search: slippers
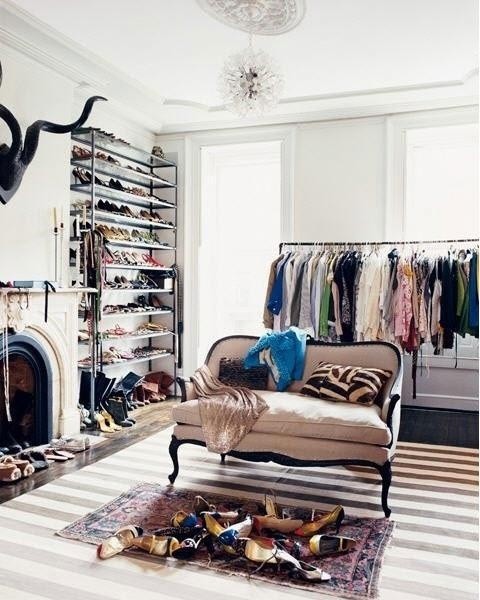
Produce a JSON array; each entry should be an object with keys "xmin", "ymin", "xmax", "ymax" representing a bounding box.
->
[{"xmin": 0, "ymin": 445, "xmax": 76, "ymax": 482}]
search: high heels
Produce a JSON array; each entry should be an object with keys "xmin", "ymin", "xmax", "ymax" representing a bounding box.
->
[
  {"xmin": 72, "ymin": 166, "xmax": 125, "ymax": 192},
  {"xmin": 131, "ymin": 487, "xmax": 357, "ymax": 582}
]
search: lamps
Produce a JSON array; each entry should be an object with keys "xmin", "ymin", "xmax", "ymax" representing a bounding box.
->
[{"xmin": 194, "ymin": 0, "xmax": 306, "ymax": 114}]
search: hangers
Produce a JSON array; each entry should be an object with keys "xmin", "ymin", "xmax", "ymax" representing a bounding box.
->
[{"xmin": 279, "ymin": 236, "xmax": 479, "ymax": 263}]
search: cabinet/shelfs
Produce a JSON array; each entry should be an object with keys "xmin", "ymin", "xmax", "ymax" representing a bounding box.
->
[{"xmin": 71, "ymin": 126, "xmax": 179, "ymax": 431}]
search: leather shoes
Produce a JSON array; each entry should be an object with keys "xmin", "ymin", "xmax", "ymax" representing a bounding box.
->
[
  {"xmin": 98, "ymin": 224, "xmax": 169, "ymax": 246},
  {"xmin": 93, "ymin": 127, "xmax": 131, "ymax": 145},
  {"xmin": 107, "ymin": 273, "xmax": 151, "ymax": 289},
  {"xmin": 98, "ymin": 199, "xmax": 166, "ymax": 224},
  {"xmin": 104, "ymin": 245, "xmax": 166, "ymax": 268}
]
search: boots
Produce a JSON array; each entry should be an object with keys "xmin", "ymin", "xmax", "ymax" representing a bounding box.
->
[{"xmin": 79, "ymin": 370, "xmax": 174, "ymax": 433}]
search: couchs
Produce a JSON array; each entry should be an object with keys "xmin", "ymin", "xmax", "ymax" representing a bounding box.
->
[{"xmin": 168, "ymin": 334, "xmax": 401, "ymax": 517}]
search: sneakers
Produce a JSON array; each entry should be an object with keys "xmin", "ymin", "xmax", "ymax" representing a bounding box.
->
[
  {"xmin": 60, "ymin": 435, "xmax": 91, "ymax": 448},
  {"xmin": 50, "ymin": 436, "xmax": 85, "ymax": 451}
]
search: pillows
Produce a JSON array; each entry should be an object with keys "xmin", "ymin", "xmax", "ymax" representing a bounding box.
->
[
  {"xmin": 300, "ymin": 361, "xmax": 392, "ymax": 407},
  {"xmin": 218, "ymin": 357, "xmax": 268, "ymax": 390}
]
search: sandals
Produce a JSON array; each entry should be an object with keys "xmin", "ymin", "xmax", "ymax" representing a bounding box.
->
[
  {"xmin": 96, "ymin": 524, "xmax": 143, "ymax": 560},
  {"xmin": 77, "ymin": 346, "xmax": 167, "ymax": 368},
  {"xmin": 71, "ymin": 145, "xmax": 109, "ymax": 162}
]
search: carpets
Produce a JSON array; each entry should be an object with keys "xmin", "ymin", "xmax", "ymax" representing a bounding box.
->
[{"xmin": 56, "ymin": 482, "xmax": 397, "ymax": 599}]
